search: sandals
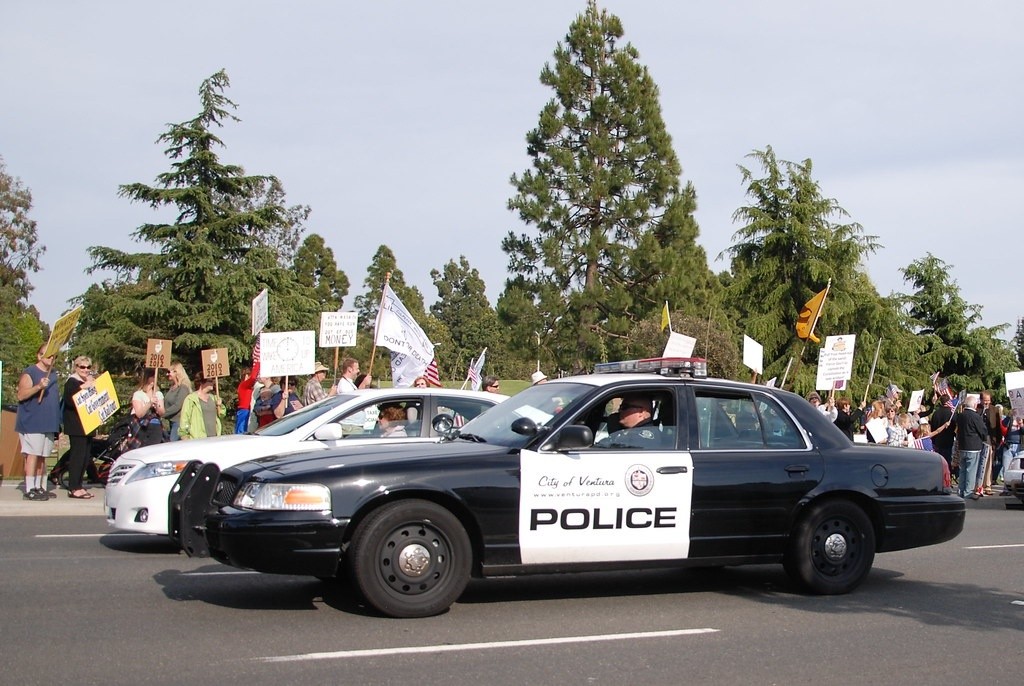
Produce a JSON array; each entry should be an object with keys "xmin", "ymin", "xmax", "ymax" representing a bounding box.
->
[{"xmin": 68, "ymin": 488, "xmax": 94, "ymax": 499}]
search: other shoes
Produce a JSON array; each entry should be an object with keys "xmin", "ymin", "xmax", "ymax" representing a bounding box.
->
[{"xmin": 959, "ymin": 482, "xmax": 1011, "ymax": 498}]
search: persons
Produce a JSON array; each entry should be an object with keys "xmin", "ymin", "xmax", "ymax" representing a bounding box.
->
[
  {"xmin": 377, "ymin": 402, "xmax": 409, "ymax": 438},
  {"xmin": 593, "ymin": 393, "xmax": 665, "ymax": 449},
  {"xmin": 480, "ymin": 371, "xmax": 565, "ymax": 417},
  {"xmin": 49, "ymin": 356, "xmax": 113, "ymax": 500},
  {"xmin": 133, "ymin": 371, "xmax": 165, "ymax": 445},
  {"xmin": 177, "ymin": 371, "xmax": 227, "ymax": 441},
  {"xmin": 154, "ymin": 361, "xmax": 194, "ymax": 442},
  {"xmin": 735, "ymin": 370, "xmax": 1024, "ymax": 501},
  {"xmin": 235, "ymin": 360, "xmax": 374, "ymax": 435},
  {"xmin": 14, "ymin": 342, "xmax": 61, "ymax": 501}
]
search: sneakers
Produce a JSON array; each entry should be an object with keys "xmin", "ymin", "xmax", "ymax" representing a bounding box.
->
[
  {"xmin": 36, "ymin": 487, "xmax": 57, "ymax": 498},
  {"xmin": 23, "ymin": 489, "xmax": 48, "ymax": 501}
]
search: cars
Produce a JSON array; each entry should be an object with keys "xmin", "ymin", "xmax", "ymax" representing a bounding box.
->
[
  {"xmin": 1004, "ymin": 451, "xmax": 1024, "ymax": 502},
  {"xmin": 97, "ymin": 388, "xmax": 609, "ymax": 536},
  {"xmin": 167, "ymin": 356, "xmax": 968, "ymax": 619}
]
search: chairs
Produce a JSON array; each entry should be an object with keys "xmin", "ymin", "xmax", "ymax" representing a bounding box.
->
[
  {"xmin": 607, "ymin": 399, "xmax": 786, "ymax": 450},
  {"xmin": 407, "ymin": 405, "xmax": 421, "ymax": 438}
]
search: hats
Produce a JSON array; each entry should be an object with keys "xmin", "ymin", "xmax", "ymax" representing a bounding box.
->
[
  {"xmin": 306, "ymin": 362, "xmax": 329, "ymax": 372},
  {"xmin": 530, "ymin": 370, "xmax": 548, "ymax": 386},
  {"xmin": 887, "ymin": 386, "xmax": 903, "ymax": 392}
]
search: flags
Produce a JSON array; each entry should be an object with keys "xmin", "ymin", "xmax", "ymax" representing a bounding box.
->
[
  {"xmin": 914, "ymin": 436, "xmax": 934, "ymax": 452},
  {"xmin": 938, "ymin": 379, "xmax": 948, "ymax": 391},
  {"xmin": 253, "ymin": 332, "xmax": 260, "ymax": 371},
  {"xmin": 929, "ymin": 372, "xmax": 941, "ymax": 383},
  {"xmin": 946, "ymin": 399, "xmax": 959, "ymax": 412},
  {"xmin": 796, "ymin": 288, "xmax": 828, "ymax": 343},
  {"xmin": 661, "ymin": 303, "xmax": 670, "ymax": 332},
  {"xmin": 375, "ymin": 280, "xmax": 486, "ymax": 428}
]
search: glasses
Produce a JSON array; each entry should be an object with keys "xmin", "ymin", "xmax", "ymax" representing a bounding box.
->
[
  {"xmin": 620, "ymin": 404, "xmax": 644, "ymax": 411},
  {"xmin": 166, "ymin": 370, "xmax": 171, "ymax": 373},
  {"xmin": 42, "ymin": 354, "xmax": 58, "ymax": 360},
  {"xmin": 77, "ymin": 364, "xmax": 92, "ymax": 369},
  {"xmin": 283, "ymin": 382, "xmax": 295, "ymax": 386},
  {"xmin": 378, "ymin": 413, "xmax": 384, "ymax": 419},
  {"xmin": 809, "ymin": 397, "xmax": 818, "ymax": 400},
  {"xmin": 490, "ymin": 385, "xmax": 499, "ymax": 388}
]
